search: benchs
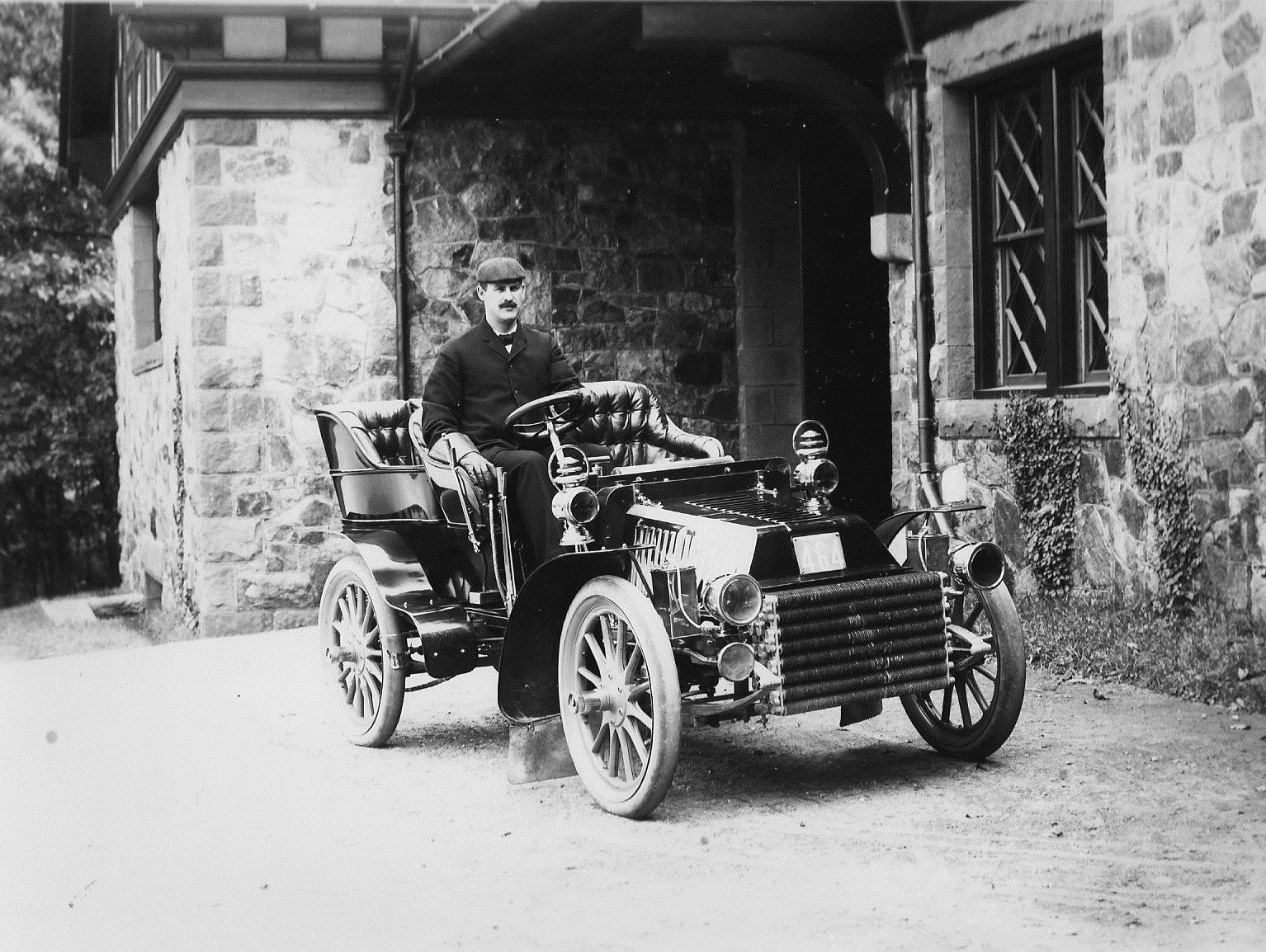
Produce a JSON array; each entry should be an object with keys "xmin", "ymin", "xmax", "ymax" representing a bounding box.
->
[{"xmin": 313, "ymin": 381, "xmax": 725, "ymax": 529}]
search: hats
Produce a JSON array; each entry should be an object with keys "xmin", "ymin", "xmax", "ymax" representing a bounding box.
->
[{"xmin": 476, "ymin": 257, "xmax": 525, "ymax": 282}]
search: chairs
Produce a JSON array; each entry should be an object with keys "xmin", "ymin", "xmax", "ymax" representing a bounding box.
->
[{"xmin": 407, "ymin": 406, "xmax": 528, "ymax": 616}]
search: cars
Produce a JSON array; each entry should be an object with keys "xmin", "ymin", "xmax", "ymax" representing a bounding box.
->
[{"xmin": 306, "ymin": 380, "xmax": 1028, "ymax": 822}]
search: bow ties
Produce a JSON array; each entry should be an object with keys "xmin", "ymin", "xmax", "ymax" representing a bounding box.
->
[{"xmin": 498, "ymin": 332, "xmax": 515, "ymax": 346}]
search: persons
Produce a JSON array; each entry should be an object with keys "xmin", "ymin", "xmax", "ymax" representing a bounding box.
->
[{"xmin": 422, "ymin": 258, "xmax": 615, "ymax": 568}]
